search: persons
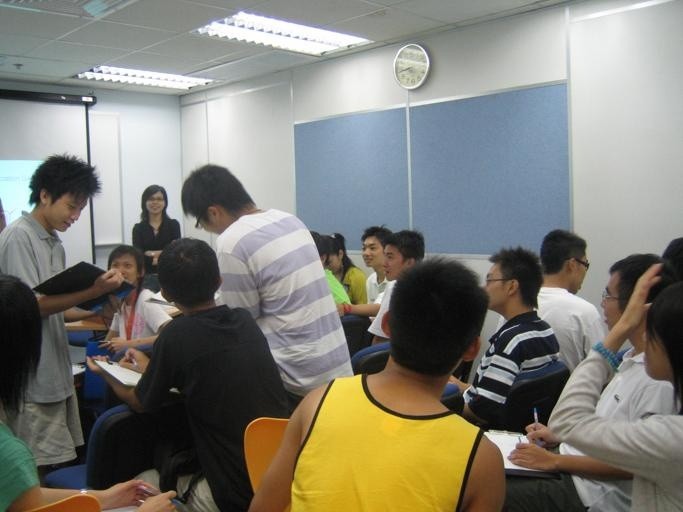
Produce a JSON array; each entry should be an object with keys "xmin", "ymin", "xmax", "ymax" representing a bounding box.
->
[
  {"xmin": 180, "ymin": 163, "xmax": 355, "ymax": 411},
  {"xmin": 132, "ymin": 184, "xmax": 182, "ymax": 297},
  {"xmin": 0, "ymin": 153, "xmax": 127, "ymax": 474},
  {"xmin": 1, "ymin": 225, "xmax": 679, "ymax": 511}
]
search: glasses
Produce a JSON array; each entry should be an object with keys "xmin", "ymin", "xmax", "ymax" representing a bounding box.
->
[
  {"xmin": 486, "ymin": 275, "xmax": 513, "ymax": 286},
  {"xmin": 602, "ymin": 290, "xmax": 618, "ymax": 302},
  {"xmin": 566, "ymin": 257, "xmax": 589, "ymax": 271}
]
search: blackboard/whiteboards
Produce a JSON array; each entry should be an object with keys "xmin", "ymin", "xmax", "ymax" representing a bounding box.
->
[{"xmin": 294, "ymin": 79, "xmax": 573, "ymax": 263}]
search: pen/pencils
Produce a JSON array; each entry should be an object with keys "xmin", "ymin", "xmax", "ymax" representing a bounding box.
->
[
  {"xmin": 138, "ymin": 486, "xmax": 182, "ymax": 505},
  {"xmin": 126, "ymin": 350, "xmax": 137, "ymax": 368},
  {"xmin": 534, "ymin": 408, "xmax": 541, "ymax": 446},
  {"xmin": 93, "ymin": 330, "xmax": 97, "ymax": 341}
]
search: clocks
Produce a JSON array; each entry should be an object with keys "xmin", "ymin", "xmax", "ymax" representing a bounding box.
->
[{"xmin": 392, "ymin": 44, "xmax": 430, "ymax": 90}]
